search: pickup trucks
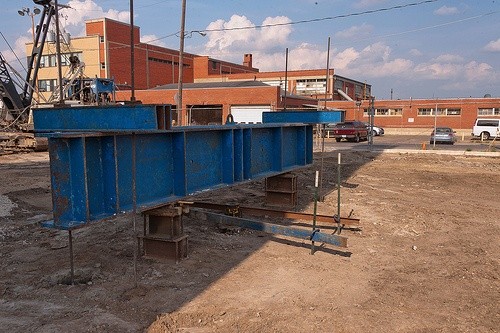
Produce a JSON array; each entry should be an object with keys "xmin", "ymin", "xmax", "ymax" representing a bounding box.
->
[{"xmin": 333, "ymin": 120, "xmax": 370, "ymax": 144}]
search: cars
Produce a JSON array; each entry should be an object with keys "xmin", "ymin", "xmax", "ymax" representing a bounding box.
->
[{"xmin": 430, "ymin": 126, "xmax": 457, "ymax": 144}]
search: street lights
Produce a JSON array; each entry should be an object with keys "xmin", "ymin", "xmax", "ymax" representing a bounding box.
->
[
  {"xmin": 17, "ymin": 6, "xmax": 43, "ymax": 109},
  {"xmin": 370, "ymin": 93, "xmax": 376, "ymax": 144}
]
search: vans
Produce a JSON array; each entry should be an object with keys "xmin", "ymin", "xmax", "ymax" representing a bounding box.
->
[{"xmin": 471, "ymin": 118, "xmax": 500, "ymax": 141}]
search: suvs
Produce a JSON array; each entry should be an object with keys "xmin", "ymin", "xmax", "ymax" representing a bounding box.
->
[{"xmin": 363, "ymin": 122, "xmax": 384, "ymax": 137}]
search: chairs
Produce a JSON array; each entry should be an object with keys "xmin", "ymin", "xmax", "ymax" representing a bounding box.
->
[
  {"xmin": 437, "ymin": 130, "xmax": 441, "ymax": 132},
  {"xmin": 446, "ymin": 130, "xmax": 449, "ymax": 132}
]
